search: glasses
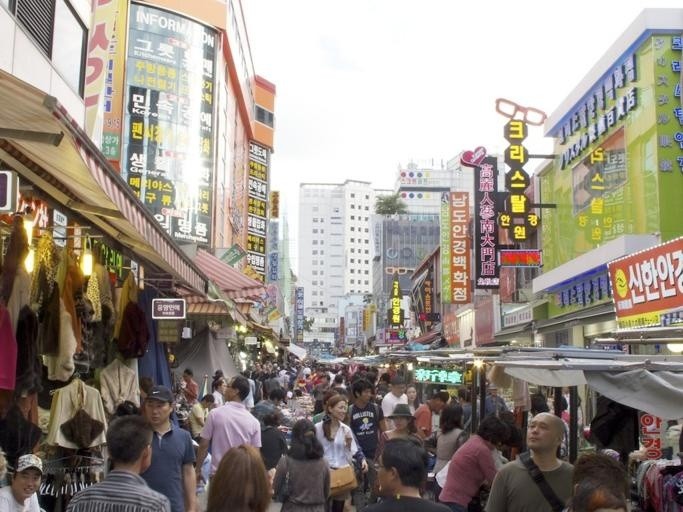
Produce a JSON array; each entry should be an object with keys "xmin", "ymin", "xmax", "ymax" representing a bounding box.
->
[
  {"xmin": 373, "ymin": 463, "xmax": 385, "ymax": 471},
  {"xmin": 223, "ymin": 384, "xmax": 232, "ymax": 388}
]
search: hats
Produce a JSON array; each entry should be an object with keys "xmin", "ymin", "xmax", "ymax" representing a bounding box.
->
[
  {"xmin": 15, "ymin": 454, "xmax": 46, "ymax": 474},
  {"xmin": 385, "ymin": 404, "xmax": 415, "ymax": 419},
  {"xmin": 488, "ymin": 382, "xmax": 495, "ymax": 390},
  {"xmin": 145, "ymin": 385, "xmax": 174, "ymax": 402},
  {"xmin": 440, "ymin": 389, "xmax": 452, "ymax": 405}
]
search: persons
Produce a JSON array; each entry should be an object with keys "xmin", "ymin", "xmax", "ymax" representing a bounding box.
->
[
  {"xmin": 196, "ymin": 375, "xmax": 262, "ymax": 493},
  {"xmin": 138, "ymin": 377, "xmax": 153, "ymax": 402},
  {"xmin": 381, "ymin": 376, "xmax": 408, "ymax": 430},
  {"xmin": 344, "ymin": 359, "xmax": 413, "ymax": 384},
  {"xmin": 250, "ymin": 387, "xmax": 284, "ymax": 428},
  {"xmin": 272, "ymin": 418, "xmax": 331, "ymax": 512},
  {"xmin": 206, "ymin": 443, "xmax": 273, "ymax": 512},
  {"xmin": 449, "ymin": 382, "xmax": 483, "ymax": 424},
  {"xmin": 1, "ymin": 455, "xmax": 43, "ymax": 511},
  {"xmin": 552, "ymin": 396, "xmax": 570, "ymax": 424},
  {"xmin": 484, "ymin": 412, "xmax": 574, "ymax": 512},
  {"xmin": 563, "ymin": 452, "xmax": 629, "ymax": 512},
  {"xmin": 138, "ymin": 385, "xmax": 196, "ymax": 512},
  {"xmin": 414, "ymin": 391, "xmax": 449, "ymax": 436},
  {"xmin": 484, "ymin": 383, "xmax": 510, "ymax": 414},
  {"xmin": 528, "ymin": 392, "xmax": 551, "ymax": 418},
  {"xmin": 589, "ymin": 415, "xmax": 623, "ymax": 464},
  {"xmin": 358, "ymin": 437, "xmax": 452, "ymax": 511},
  {"xmin": 314, "ymin": 395, "xmax": 357, "ymax": 512},
  {"xmin": 259, "ymin": 407, "xmax": 290, "ymax": 474},
  {"xmin": 347, "ymin": 381, "xmax": 387, "ymax": 512},
  {"xmin": 234, "ymin": 354, "xmax": 351, "ymax": 394},
  {"xmin": 191, "ymin": 394, "xmax": 215, "ymax": 444},
  {"xmin": 67, "ymin": 415, "xmax": 171, "ymax": 512},
  {"xmin": 115, "ymin": 401, "xmax": 139, "ymax": 419},
  {"xmin": 405, "ymin": 383, "xmax": 418, "ymax": 415},
  {"xmin": 375, "ymin": 404, "xmax": 425, "ymax": 465},
  {"xmin": 212, "ymin": 368, "xmax": 225, "ymax": 395},
  {"xmin": 181, "ymin": 368, "xmax": 200, "ymax": 409},
  {"xmin": 438, "ymin": 415, "xmax": 511, "ymax": 512},
  {"xmin": 212, "ymin": 377, "xmax": 226, "ymax": 409},
  {"xmin": 431, "ymin": 402, "xmax": 469, "ymax": 473}
]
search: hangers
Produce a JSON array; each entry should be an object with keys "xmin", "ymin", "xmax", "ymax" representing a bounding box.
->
[
  {"xmin": 73, "ymin": 372, "xmax": 81, "ymax": 379},
  {"xmin": 39, "ymin": 465, "xmax": 106, "ymax": 497}
]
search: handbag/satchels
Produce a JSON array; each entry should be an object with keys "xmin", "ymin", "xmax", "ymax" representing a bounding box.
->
[
  {"xmin": 270, "ymin": 459, "xmax": 291, "ymax": 506},
  {"xmin": 521, "ymin": 451, "xmax": 562, "ymax": 512},
  {"xmin": 325, "ymin": 464, "xmax": 358, "ymax": 494}
]
